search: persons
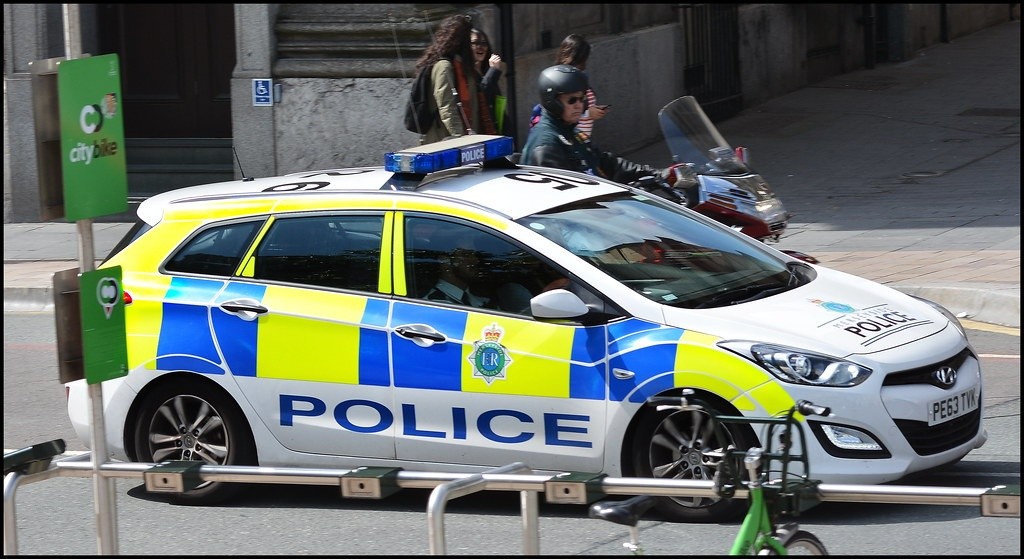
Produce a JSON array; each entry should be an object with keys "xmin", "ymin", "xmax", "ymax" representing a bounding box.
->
[
  {"xmin": 529, "ymin": 34, "xmax": 610, "ymax": 145},
  {"xmin": 418, "ymin": 229, "xmax": 569, "ymax": 315},
  {"xmin": 417, "ymin": 14, "xmax": 479, "ymax": 145},
  {"xmin": 519, "ymin": 64, "xmax": 695, "ymax": 195},
  {"xmin": 469, "ymin": 28, "xmax": 505, "ymax": 138}
]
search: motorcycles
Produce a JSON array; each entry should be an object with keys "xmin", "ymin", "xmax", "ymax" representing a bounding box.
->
[{"xmin": 626, "ymin": 96, "xmax": 821, "ymax": 264}]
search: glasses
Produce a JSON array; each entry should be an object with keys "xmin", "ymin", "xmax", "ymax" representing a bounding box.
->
[
  {"xmin": 560, "ymin": 94, "xmax": 587, "ymax": 105},
  {"xmin": 472, "ymin": 41, "xmax": 487, "ymax": 46}
]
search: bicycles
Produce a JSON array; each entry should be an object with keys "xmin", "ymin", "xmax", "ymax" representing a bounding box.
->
[{"xmin": 585, "ymin": 389, "xmax": 830, "ymax": 556}]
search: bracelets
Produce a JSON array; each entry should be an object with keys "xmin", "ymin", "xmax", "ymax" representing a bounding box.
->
[{"xmin": 584, "ymin": 110, "xmax": 590, "ymax": 119}]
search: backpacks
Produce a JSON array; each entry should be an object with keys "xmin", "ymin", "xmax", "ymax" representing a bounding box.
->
[{"xmin": 405, "ymin": 63, "xmax": 442, "ymax": 134}]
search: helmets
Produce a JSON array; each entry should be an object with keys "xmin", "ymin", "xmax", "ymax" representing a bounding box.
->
[{"xmin": 538, "ymin": 64, "xmax": 590, "ymax": 119}]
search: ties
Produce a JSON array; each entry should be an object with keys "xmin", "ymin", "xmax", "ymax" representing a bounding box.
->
[{"xmin": 462, "ymin": 292, "xmax": 469, "ymax": 304}]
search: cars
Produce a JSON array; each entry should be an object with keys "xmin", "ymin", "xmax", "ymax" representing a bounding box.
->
[{"xmin": 64, "ymin": 133, "xmax": 989, "ymax": 525}]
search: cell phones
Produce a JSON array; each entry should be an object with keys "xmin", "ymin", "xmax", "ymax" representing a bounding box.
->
[{"xmin": 602, "ymin": 104, "xmax": 611, "ymax": 110}]
